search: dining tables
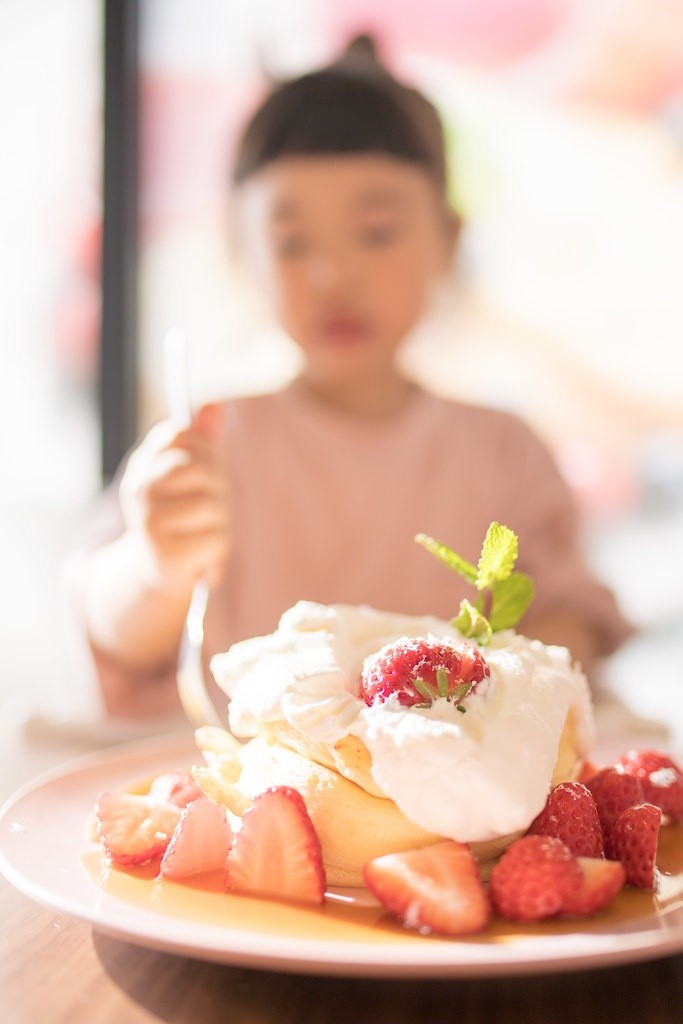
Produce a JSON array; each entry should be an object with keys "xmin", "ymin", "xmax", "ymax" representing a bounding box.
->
[{"xmin": 0, "ymin": 717, "xmax": 683, "ymax": 1024}]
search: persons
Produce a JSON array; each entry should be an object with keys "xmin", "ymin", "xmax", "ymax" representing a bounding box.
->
[{"xmin": 73, "ymin": 42, "xmax": 631, "ymax": 720}]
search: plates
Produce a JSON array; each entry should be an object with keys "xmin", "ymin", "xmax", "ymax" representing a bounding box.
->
[{"xmin": 0, "ymin": 730, "xmax": 681, "ymax": 978}]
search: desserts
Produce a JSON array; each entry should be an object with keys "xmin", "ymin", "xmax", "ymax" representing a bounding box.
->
[{"xmin": 188, "ymin": 519, "xmax": 597, "ymax": 886}]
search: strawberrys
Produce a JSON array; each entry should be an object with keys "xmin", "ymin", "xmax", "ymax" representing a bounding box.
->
[
  {"xmin": 361, "ymin": 750, "xmax": 682, "ymax": 935},
  {"xmin": 89, "ymin": 772, "xmax": 326, "ymax": 906}
]
